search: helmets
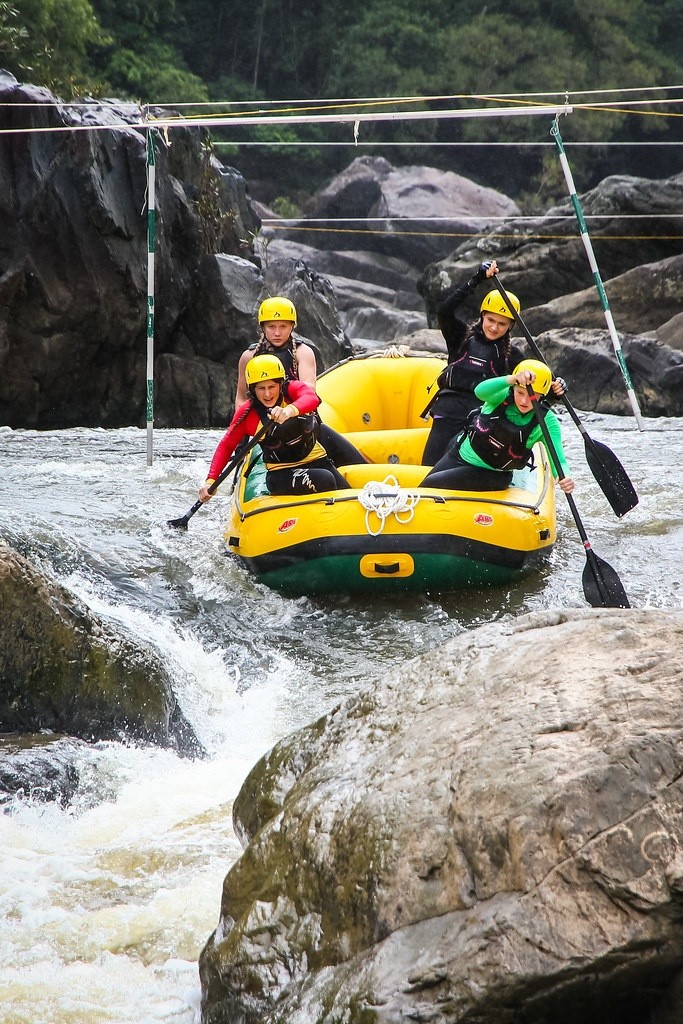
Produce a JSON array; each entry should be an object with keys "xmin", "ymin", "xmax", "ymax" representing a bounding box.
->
[
  {"xmin": 245, "ymin": 354, "xmax": 285, "ymax": 388},
  {"xmin": 512, "ymin": 359, "xmax": 552, "ymax": 396},
  {"xmin": 258, "ymin": 297, "xmax": 297, "ymax": 325},
  {"xmin": 480, "ymin": 289, "xmax": 520, "ymax": 320}
]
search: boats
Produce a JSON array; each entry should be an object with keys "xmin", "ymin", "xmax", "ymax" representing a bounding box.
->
[{"xmin": 220, "ymin": 344, "xmax": 566, "ymax": 595}]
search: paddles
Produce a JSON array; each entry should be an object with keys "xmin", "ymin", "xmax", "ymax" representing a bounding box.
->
[
  {"xmin": 524, "ymin": 382, "xmax": 630, "ymax": 607},
  {"xmin": 166, "ymin": 419, "xmax": 274, "ymax": 530},
  {"xmin": 490, "ymin": 269, "xmax": 639, "ymax": 518},
  {"xmin": 228, "ymin": 433, "xmax": 250, "ymax": 494}
]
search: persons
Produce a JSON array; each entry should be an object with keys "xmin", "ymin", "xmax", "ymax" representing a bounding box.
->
[
  {"xmin": 197, "ymin": 356, "xmax": 353, "ymax": 503},
  {"xmin": 419, "ymin": 261, "xmax": 569, "ymax": 468},
  {"xmin": 233, "ymin": 296, "xmax": 367, "ymax": 468},
  {"xmin": 416, "ymin": 358, "xmax": 576, "ymax": 494}
]
